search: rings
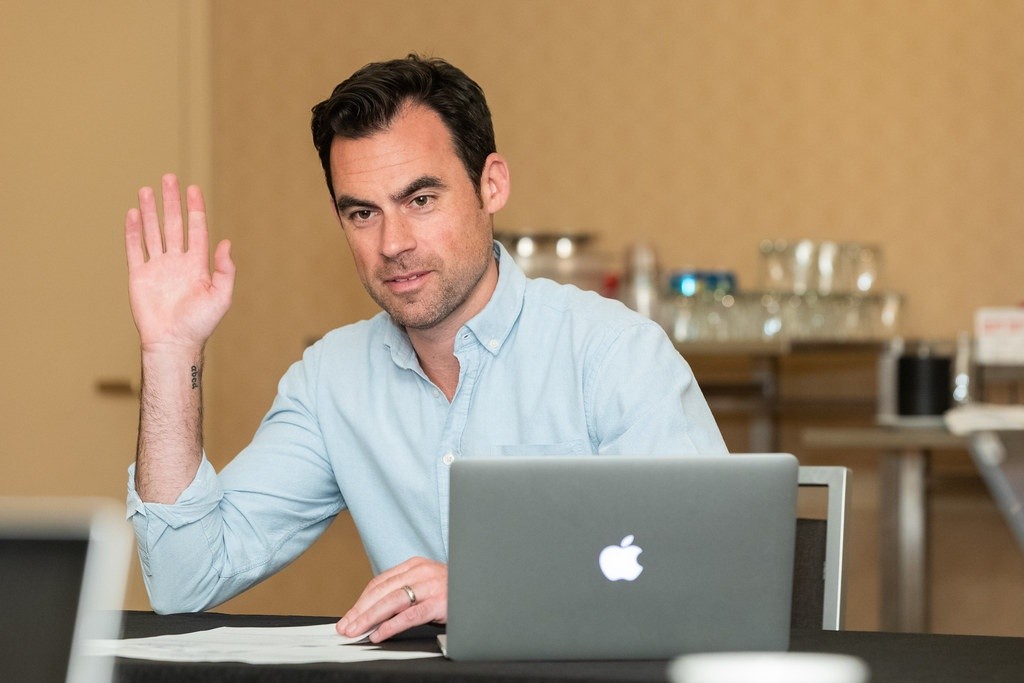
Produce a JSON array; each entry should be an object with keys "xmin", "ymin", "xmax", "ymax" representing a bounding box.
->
[{"xmin": 400, "ymin": 584, "xmax": 417, "ymax": 607}]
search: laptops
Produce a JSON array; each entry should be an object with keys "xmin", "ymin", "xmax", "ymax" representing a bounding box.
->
[{"xmin": 439, "ymin": 452, "xmax": 798, "ymax": 661}]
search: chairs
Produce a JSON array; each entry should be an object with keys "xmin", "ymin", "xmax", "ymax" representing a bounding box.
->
[{"xmin": 788, "ymin": 465, "xmax": 854, "ymax": 632}]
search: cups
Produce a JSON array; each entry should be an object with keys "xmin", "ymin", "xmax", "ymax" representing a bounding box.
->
[{"xmin": 898, "ymin": 354, "xmax": 952, "ymax": 416}]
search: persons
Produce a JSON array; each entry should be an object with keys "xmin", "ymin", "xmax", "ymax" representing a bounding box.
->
[{"xmin": 123, "ymin": 53, "xmax": 732, "ymax": 644}]
